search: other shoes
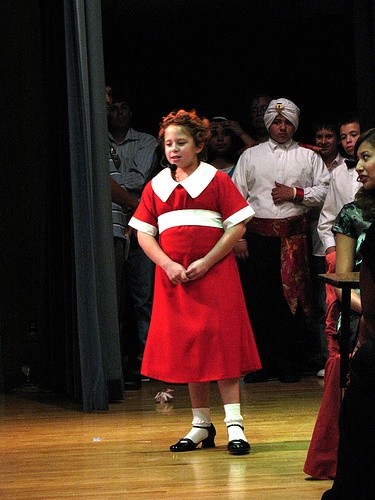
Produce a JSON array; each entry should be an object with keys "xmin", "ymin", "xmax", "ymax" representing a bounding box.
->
[
  {"xmin": 311, "ymin": 362, "xmax": 327, "ymax": 379},
  {"xmin": 322, "ymin": 489, "xmax": 339, "ymax": 500},
  {"xmin": 277, "ymin": 366, "xmax": 297, "ymax": 383},
  {"xmin": 244, "ymin": 368, "xmax": 278, "ymax": 382}
]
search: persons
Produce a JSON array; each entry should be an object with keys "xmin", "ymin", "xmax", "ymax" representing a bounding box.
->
[{"xmin": 96, "ymin": 82, "xmax": 374, "ymax": 500}]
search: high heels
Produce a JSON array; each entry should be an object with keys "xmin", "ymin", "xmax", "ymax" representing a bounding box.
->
[
  {"xmin": 227, "ymin": 424, "xmax": 250, "ymax": 453},
  {"xmin": 169, "ymin": 423, "xmax": 216, "ymax": 453}
]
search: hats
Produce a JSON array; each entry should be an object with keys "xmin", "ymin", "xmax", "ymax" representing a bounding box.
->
[{"xmin": 263, "ymin": 98, "xmax": 300, "ymax": 133}]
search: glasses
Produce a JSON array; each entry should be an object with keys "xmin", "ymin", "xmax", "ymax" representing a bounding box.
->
[{"xmin": 249, "ymin": 103, "xmax": 267, "ymax": 113}]
